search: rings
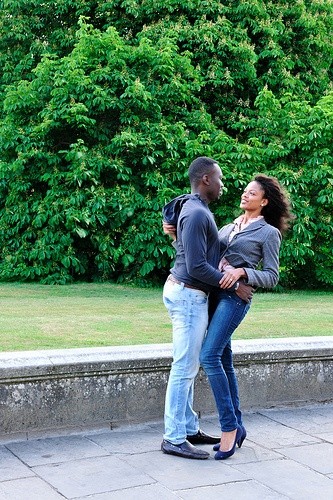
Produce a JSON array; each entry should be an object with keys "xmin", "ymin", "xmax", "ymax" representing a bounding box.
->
[{"xmin": 230, "ymin": 279, "xmax": 233, "ymax": 282}]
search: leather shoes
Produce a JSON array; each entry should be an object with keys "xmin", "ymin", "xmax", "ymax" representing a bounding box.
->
[
  {"xmin": 160, "ymin": 438, "xmax": 210, "ymax": 460},
  {"xmin": 188, "ymin": 432, "xmax": 222, "ymax": 444}
]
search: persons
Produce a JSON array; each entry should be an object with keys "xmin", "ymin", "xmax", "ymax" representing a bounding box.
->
[
  {"xmin": 161, "ymin": 172, "xmax": 296, "ymax": 462},
  {"xmin": 160, "ymin": 155, "xmax": 256, "ymax": 461}
]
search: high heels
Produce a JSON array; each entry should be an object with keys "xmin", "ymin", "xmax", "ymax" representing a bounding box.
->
[
  {"xmin": 214, "ymin": 425, "xmax": 242, "ymax": 459},
  {"xmin": 214, "ymin": 424, "xmax": 246, "ymax": 449}
]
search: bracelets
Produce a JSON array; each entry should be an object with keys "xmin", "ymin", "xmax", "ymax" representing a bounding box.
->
[{"xmin": 235, "ymin": 282, "xmax": 239, "ymax": 290}]
